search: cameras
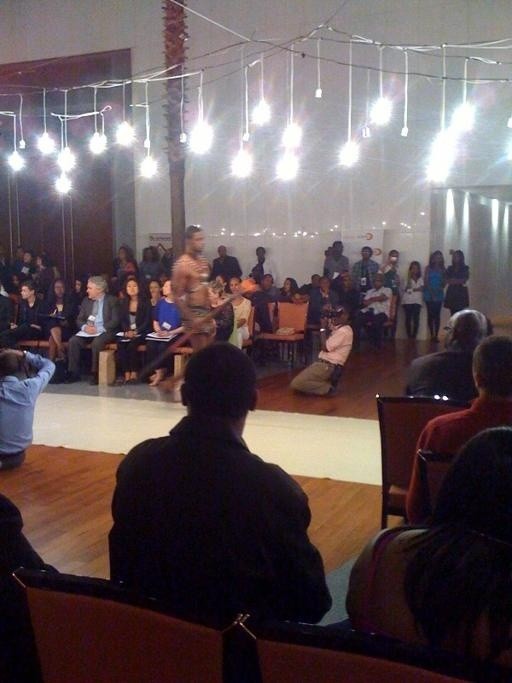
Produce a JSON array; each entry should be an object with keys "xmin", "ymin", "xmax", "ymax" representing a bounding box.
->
[{"xmin": 320, "ymin": 310, "xmax": 338, "ymax": 318}]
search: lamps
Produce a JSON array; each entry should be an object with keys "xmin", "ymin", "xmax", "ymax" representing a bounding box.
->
[{"xmin": 0, "ymin": 5, "xmax": 512, "ymax": 195}]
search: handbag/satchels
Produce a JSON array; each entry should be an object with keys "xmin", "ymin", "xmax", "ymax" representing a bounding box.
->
[{"xmin": 48, "ymin": 358, "xmax": 68, "ymax": 383}]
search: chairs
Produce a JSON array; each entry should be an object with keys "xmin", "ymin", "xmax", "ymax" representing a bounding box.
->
[
  {"xmin": 376, "ymin": 392, "xmax": 473, "ymax": 533},
  {"xmin": 415, "ymin": 449, "xmax": 456, "ymax": 522},
  {"xmin": 243, "ymin": 607, "xmax": 512, "ymax": 683},
  {"xmin": 2, "ymin": 273, "xmax": 398, "ymax": 388},
  {"xmin": 11, "ymin": 562, "xmax": 243, "ymax": 682}
]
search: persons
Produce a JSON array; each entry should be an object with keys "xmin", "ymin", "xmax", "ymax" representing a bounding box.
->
[
  {"xmin": 209, "ymin": 242, "xmax": 404, "ymax": 351},
  {"xmin": 0, "ymin": 246, "xmax": 184, "ymax": 385},
  {"xmin": 444, "ymin": 250, "xmax": 468, "ymax": 316},
  {"xmin": 0, "ymin": 490, "xmax": 61, "ymax": 683},
  {"xmin": 405, "ymin": 335, "xmax": 512, "ymax": 526},
  {"xmin": 157, "ymin": 226, "xmax": 217, "ymax": 396},
  {"xmin": 0, "ymin": 347, "xmax": 56, "ymax": 469},
  {"xmin": 405, "ymin": 307, "xmax": 492, "ymax": 400},
  {"xmin": 343, "ymin": 426, "xmax": 512, "ymax": 682},
  {"xmin": 402, "ymin": 261, "xmax": 423, "ymax": 341},
  {"xmin": 422, "ymin": 251, "xmax": 447, "ymax": 342},
  {"xmin": 109, "ymin": 340, "xmax": 333, "ymax": 637},
  {"xmin": 290, "ymin": 305, "xmax": 353, "ymax": 397}
]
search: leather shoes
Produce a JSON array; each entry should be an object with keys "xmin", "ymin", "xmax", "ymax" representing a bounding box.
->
[{"xmin": 123, "ymin": 374, "xmax": 165, "ymax": 385}]
